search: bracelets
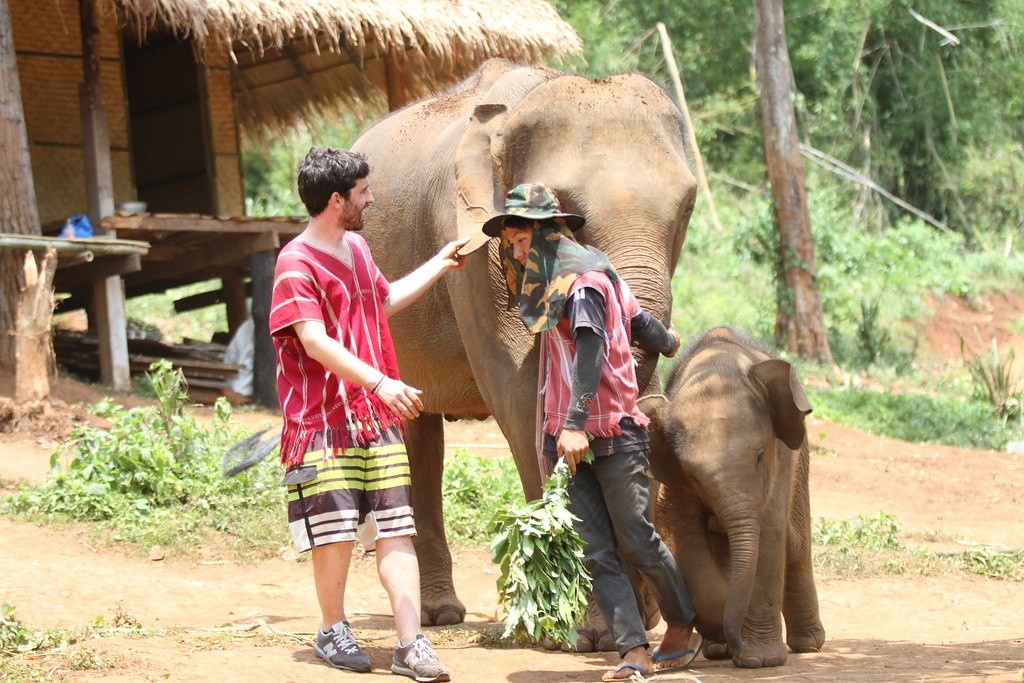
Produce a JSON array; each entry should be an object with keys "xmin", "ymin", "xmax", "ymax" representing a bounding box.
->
[{"xmin": 371, "ymin": 375, "xmax": 386, "ymax": 395}]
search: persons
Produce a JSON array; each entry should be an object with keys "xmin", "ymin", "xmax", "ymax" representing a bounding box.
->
[
  {"xmin": 270, "ymin": 147, "xmax": 470, "ymax": 681},
  {"xmin": 481, "ymin": 185, "xmax": 702, "ymax": 682}
]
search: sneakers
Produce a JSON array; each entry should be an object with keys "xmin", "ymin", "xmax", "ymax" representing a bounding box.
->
[
  {"xmin": 314, "ymin": 618, "xmax": 372, "ymax": 672},
  {"xmin": 390, "ymin": 632, "xmax": 450, "ymax": 682}
]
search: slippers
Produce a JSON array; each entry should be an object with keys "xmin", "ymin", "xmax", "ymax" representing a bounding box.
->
[
  {"xmin": 602, "ymin": 663, "xmax": 656, "ymax": 681},
  {"xmin": 649, "ymin": 632, "xmax": 703, "ymax": 673}
]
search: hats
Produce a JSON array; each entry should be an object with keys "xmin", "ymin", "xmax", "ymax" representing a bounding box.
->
[{"xmin": 482, "ymin": 182, "xmax": 586, "ymax": 237}]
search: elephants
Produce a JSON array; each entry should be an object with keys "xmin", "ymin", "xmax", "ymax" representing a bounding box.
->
[{"xmin": 347, "ymin": 58, "xmax": 825, "ymax": 667}]
